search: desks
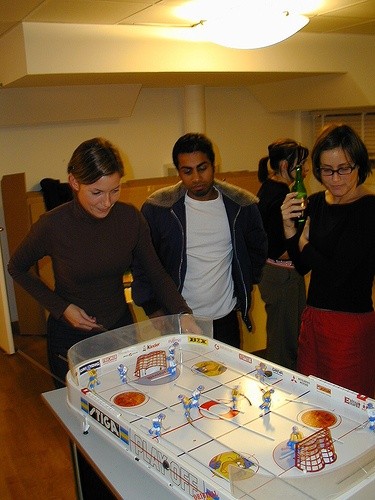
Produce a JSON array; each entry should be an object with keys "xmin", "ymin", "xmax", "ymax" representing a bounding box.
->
[{"xmin": 41, "ymin": 371, "xmax": 374, "ymax": 499}]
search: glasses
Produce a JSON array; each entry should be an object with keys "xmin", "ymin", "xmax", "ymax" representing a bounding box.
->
[{"xmin": 318, "ymin": 164, "xmax": 358, "ymax": 175}]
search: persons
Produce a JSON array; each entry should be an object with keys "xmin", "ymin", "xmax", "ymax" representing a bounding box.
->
[
  {"xmin": 177, "ymin": 394, "xmax": 196, "ymax": 421},
  {"xmin": 365, "ymin": 403, "xmax": 375, "ymax": 434},
  {"xmin": 166, "ymin": 342, "xmax": 180, "ymax": 360},
  {"xmin": 259, "ymin": 387, "xmax": 275, "ymax": 412},
  {"xmin": 146, "ymin": 411, "xmax": 173, "ymax": 444},
  {"xmin": 280, "ymin": 120, "xmax": 375, "ymax": 400},
  {"xmin": 6, "ymin": 134, "xmax": 206, "ymax": 500},
  {"xmin": 246, "ymin": 137, "xmax": 309, "ymax": 376},
  {"xmin": 231, "ymin": 384, "xmax": 241, "ymax": 414},
  {"xmin": 285, "ymin": 424, "xmax": 306, "ymax": 451},
  {"xmin": 167, "ymin": 355, "xmax": 178, "ymax": 376},
  {"xmin": 87, "ymin": 367, "xmax": 102, "ymax": 394},
  {"xmin": 128, "ymin": 129, "xmax": 266, "ymax": 352},
  {"xmin": 189, "ymin": 385, "xmax": 204, "ymax": 413},
  {"xmin": 115, "ymin": 362, "xmax": 129, "ymax": 385}
]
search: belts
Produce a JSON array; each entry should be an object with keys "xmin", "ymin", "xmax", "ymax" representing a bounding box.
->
[{"xmin": 267, "ymin": 258, "xmax": 294, "ymax": 268}]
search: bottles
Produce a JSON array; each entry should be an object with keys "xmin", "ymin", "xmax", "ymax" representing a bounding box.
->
[{"xmin": 290, "ymin": 165, "xmax": 308, "ymax": 222}]
